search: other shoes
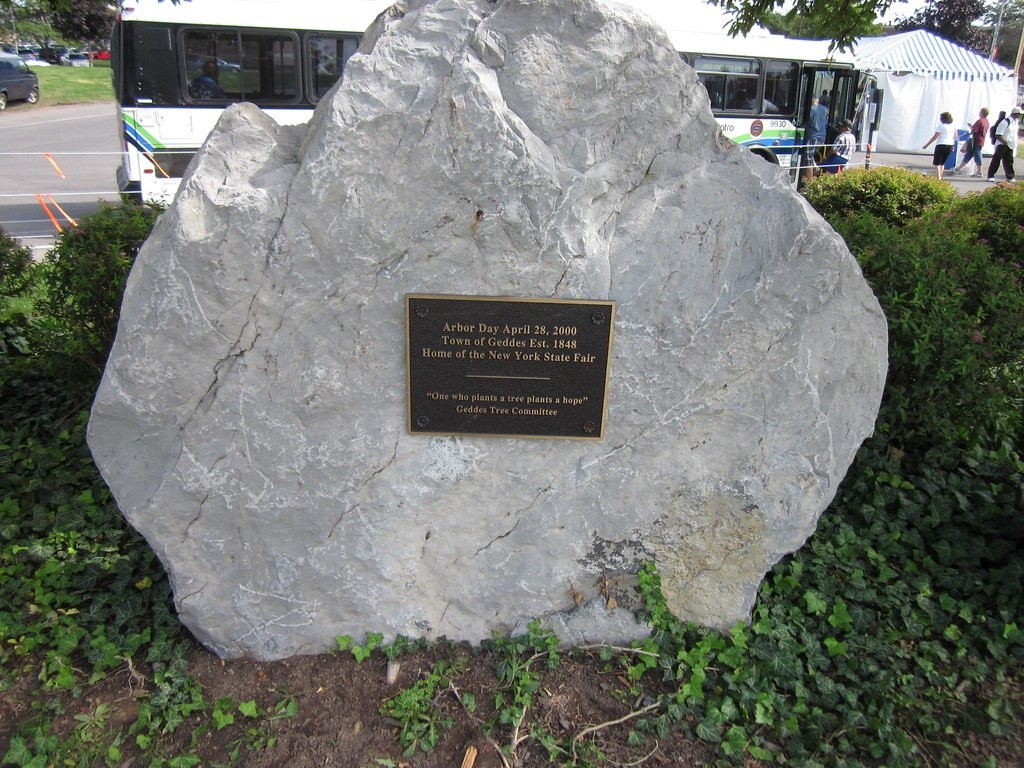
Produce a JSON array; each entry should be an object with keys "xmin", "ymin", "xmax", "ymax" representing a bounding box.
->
[
  {"xmin": 987, "ymin": 177, "xmax": 994, "ymax": 182},
  {"xmin": 1006, "ymin": 178, "xmax": 1016, "ymax": 182},
  {"xmin": 813, "ymin": 168, "xmax": 820, "ymax": 176},
  {"xmin": 970, "ymin": 171, "xmax": 983, "ymax": 177},
  {"xmin": 950, "ymin": 167, "xmax": 959, "ymax": 175}
]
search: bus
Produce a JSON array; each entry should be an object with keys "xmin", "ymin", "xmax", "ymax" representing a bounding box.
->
[{"xmin": 107, "ymin": 0, "xmax": 887, "ymax": 226}]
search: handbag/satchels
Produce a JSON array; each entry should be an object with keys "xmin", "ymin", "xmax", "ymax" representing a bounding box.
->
[
  {"xmin": 968, "ymin": 137, "xmax": 974, "ymax": 152},
  {"xmin": 960, "ymin": 141, "xmax": 968, "ymax": 153}
]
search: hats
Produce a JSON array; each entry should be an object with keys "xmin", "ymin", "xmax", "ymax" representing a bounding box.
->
[
  {"xmin": 837, "ymin": 119, "xmax": 854, "ymax": 131},
  {"xmin": 1012, "ymin": 107, "xmax": 1024, "ymax": 114}
]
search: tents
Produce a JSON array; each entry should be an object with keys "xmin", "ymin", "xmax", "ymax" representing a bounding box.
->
[{"xmin": 826, "ymin": 29, "xmax": 1019, "ymax": 154}]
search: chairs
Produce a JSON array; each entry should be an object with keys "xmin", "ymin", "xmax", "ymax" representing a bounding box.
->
[
  {"xmin": 734, "ymin": 90, "xmax": 749, "ymax": 109},
  {"xmin": 188, "ymin": 77, "xmax": 227, "ymax": 101}
]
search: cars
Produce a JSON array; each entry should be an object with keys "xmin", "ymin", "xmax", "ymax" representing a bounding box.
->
[
  {"xmin": 0, "ymin": 53, "xmax": 40, "ymax": 110},
  {"xmin": 2, "ymin": 42, "xmax": 92, "ymax": 68},
  {"xmin": 93, "ymin": 49, "xmax": 111, "ymax": 60}
]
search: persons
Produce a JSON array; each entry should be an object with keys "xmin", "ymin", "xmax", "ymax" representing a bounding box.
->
[
  {"xmin": 819, "ymin": 89, "xmax": 840, "ymax": 109},
  {"xmin": 954, "ymin": 107, "xmax": 989, "ymax": 178},
  {"xmin": 922, "ymin": 112, "xmax": 957, "ymax": 180},
  {"xmin": 820, "ymin": 119, "xmax": 857, "ymax": 174},
  {"xmin": 746, "ymin": 87, "xmax": 780, "ymax": 114},
  {"xmin": 987, "ymin": 107, "xmax": 1024, "ymax": 183},
  {"xmin": 188, "ymin": 60, "xmax": 226, "ymax": 99},
  {"xmin": 804, "ymin": 93, "xmax": 827, "ymax": 180}
]
